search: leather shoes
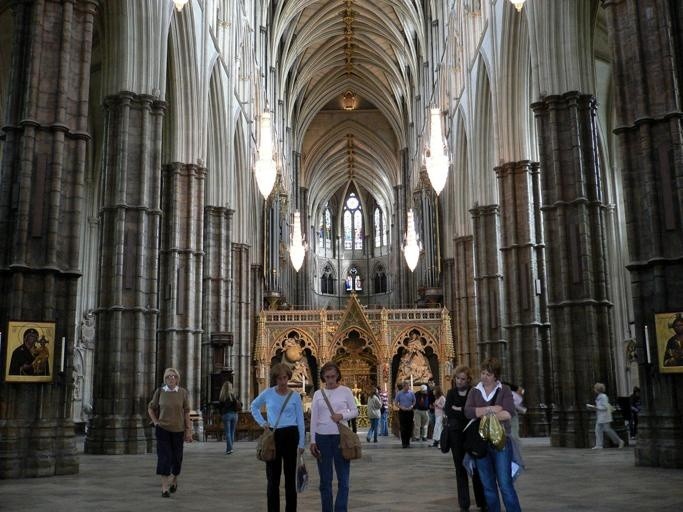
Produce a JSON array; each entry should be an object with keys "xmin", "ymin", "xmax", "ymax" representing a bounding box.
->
[{"xmin": 161, "ymin": 482, "xmax": 178, "ymax": 497}]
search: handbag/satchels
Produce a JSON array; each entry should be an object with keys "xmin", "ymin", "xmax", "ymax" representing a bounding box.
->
[
  {"xmin": 255, "ymin": 430, "xmax": 275, "ymax": 462},
  {"xmin": 463, "ymin": 434, "xmax": 489, "ymax": 458},
  {"xmin": 148, "ymin": 403, "xmax": 159, "ymax": 427},
  {"xmin": 479, "ymin": 407, "xmax": 505, "ymax": 449},
  {"xmin": 338, "ymin": 423, "xmax": 362, "ymax": 461},
  {"xmin": 608, "ymin": 403, "xmax": 616, "ymax": 413},
  {"xmin": 440, "ymin": 428, "xmax": 451, "ymax": 454},
  {"xmin": 297, "ymin": 456, "xmax": 308, "ymax": 493}
]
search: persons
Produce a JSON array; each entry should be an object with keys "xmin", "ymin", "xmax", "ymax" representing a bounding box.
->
[
  {"xmin": 462, "ymin": 359, "xmax": 522, "ymax": 511},
  {"xmin": 509, "ymin": 383, "xmax": 527, "ymax": 440},
  {"xmin": 663, "ymin": 316, "xmax": 683, "ymax": 367},
  {"xmin": 8, "ymin": 329, "xmax": 48, "ymax": 376},
  {"xmin": 626, "ymin": 385, "xmax": 640, "ymax": 440},
  {"xmin": 379, "ymin": 385, "xmax": 388, "ymax": 436},
  {"xmin": 347, "ymin": 389, "xmax": 361, "ymax": 434},
  {"xmin": 310, "ymin": 361, "xmax": 358, "ymax": 511},
  {"xmin": 393, "ymin": 377, "xmax": 447, "ymax": 449},
  {"xmin": 365, "ymin": 387, "xmax": 382, "ymax": 443},
  {"xmin": 248, "ymin": 363, "xmax": 306, "ymax": 512},
  {"xmin": 218, "ymin": 381, "xmax": 241, "ymax": 454},
  {"xmin": 146, "ymin": 367, "xmax": 194, "ymax": 498},
  {"xmin": 585, "ymin": 383, "xmax": 625, "ymax": 450},
  {"xmin": 444, "ymin": 365, "xmax": 484, "ymax": 511}
]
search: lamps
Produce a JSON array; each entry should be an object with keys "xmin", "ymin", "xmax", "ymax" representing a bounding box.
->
[
  {"xmin": 290, "ymin": 212, "xmax": 305, "ymax": 274},
  {"xmin": 255, "ymin": 112, "xmax": 277, "ymax": 199},
  {"xmin": 511, "ymin": 0, "xmax": 525, "ymax": 11},
  {"xmin": 174, "ymin": 0, "xmax": 188, "ymax": 9},
  {"xmin": 343, "ymin": 96, "xmax": 356, "ymax": 111},
  {"xmin": 173, "ymin": 0, "xmax": 527, "ymax": 275},
  {"xmin": 426, "ymin": 108, "xmax": 450, "ymax": 196},
  {"xmin": 404, "ymin": 212, "xmax": 419, "ymax": 272}
]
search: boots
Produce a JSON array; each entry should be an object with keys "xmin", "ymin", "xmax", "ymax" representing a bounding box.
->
[{"xmin": 429, "ymin": 438, "xmax": 439, "ymax": 448}]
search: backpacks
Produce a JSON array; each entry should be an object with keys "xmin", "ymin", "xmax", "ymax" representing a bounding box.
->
[{"xmin": 231, "ymin": 394, "xmax": 244, "ymax": 412}]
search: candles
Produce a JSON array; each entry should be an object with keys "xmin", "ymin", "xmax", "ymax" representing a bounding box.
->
[
  {"xmin": 302, "ymin": 375, "xmax": 305, "ymax": 392},
  {"xmin": 644, "ymin": 325, "xmax": 651, "ymax": 363},
  {"xmin": 410, "ymin": 374, "xmax": 413, "ymax": 391},
  {"xmin": 60, "ymin": 337, "xmax": 65, "ymax": 372},
  {"xmin": 384, "ymin": 383, "xmax": 386, "ymax": 391}
]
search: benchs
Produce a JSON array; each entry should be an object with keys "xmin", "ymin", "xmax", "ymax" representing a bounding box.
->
[{"xmin": 214, "ymin": 411, "xmax": 309, "ymax": 442}]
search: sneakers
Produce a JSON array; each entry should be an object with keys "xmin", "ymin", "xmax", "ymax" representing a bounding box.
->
[
  {"xmin": 618, "ymin": 439, "xmax": 624, "ymax": 449},
  {"xmin": 377, "ymin": 432, "xmax": 388, "ymax": 436},
  {"xmin": 403, "ymin": 436, "xmax": 427, "ymax": 448},
  {"xmin": 591, "ymin": 444, "xmax": 603, "ymax": 450},
  {"xmin": 366, "ymin": 437, "xmax": 380, "ymax": 443},
  {"xmin": 224, "ymin": 449, "xmax": 234, "ymax": 455}
]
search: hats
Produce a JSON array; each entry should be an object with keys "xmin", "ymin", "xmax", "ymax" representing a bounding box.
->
[
  {"xmin": 594, "ymin": 383, "xmax": 605, "ymax": 392},
  {"xmin": 24, "ymin": 329, "xmax": 39, "ymax": 341},
  {"xmin": 420, "ymin": 385, "xmax": 428, "ymax": 391}
]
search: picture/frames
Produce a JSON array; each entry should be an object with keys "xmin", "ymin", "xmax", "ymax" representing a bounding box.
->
[
  {"xmin": 3, "ymin": 320, "xmax": 56, "ymax": 384},
  {"xmin": 654, "ymin": 311, "xmax": 682, "ymax": 374}
]
